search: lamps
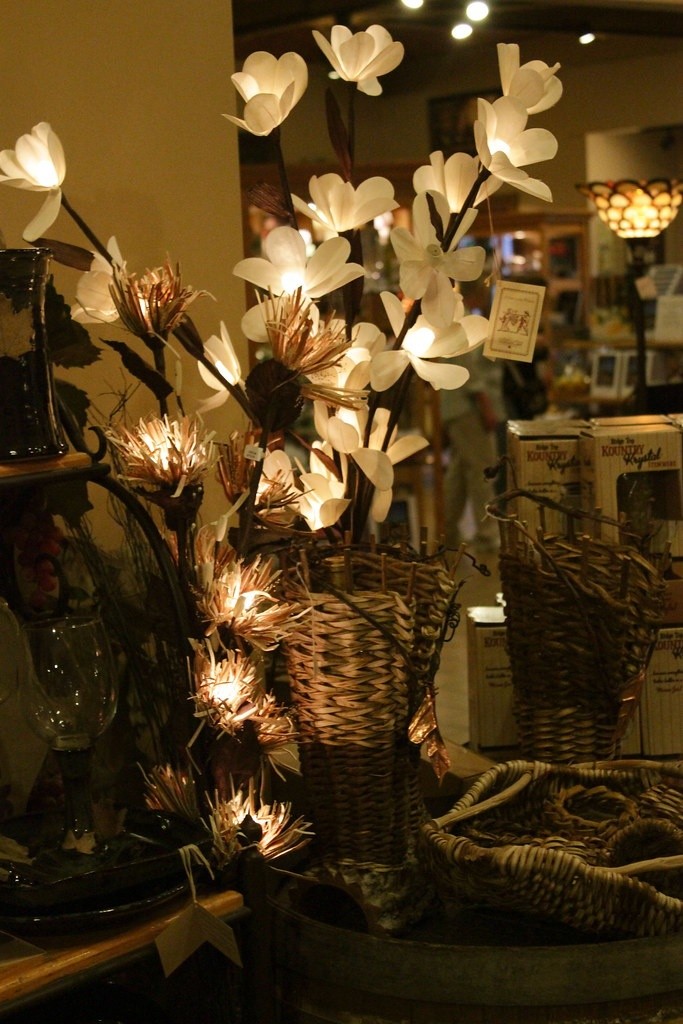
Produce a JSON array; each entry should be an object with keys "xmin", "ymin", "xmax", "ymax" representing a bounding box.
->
[{"xmin": 578, "ymin": 179, "xmax": 683, "ymax": 414}]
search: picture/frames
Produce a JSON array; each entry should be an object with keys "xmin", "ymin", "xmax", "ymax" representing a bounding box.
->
[{"xmin": 427, "ymin": 88, "xmax": 507, "ymax": 158}]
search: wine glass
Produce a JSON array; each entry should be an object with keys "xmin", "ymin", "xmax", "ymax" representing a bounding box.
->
[{"xmin": 17, "ymin": 616, "xmax": 118, "ymax": 875}]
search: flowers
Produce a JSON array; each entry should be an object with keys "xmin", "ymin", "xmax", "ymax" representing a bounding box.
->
[{"xmin": 2, "ymin": 16, "xmax": 592, "ymax": 584}]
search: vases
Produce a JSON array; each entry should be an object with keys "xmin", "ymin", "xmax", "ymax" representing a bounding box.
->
[{"xmin": 266, "ymin": 547, "xmax": 466, "ymax": 887}]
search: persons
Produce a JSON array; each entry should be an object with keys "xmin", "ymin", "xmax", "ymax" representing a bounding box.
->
[{"xmin": 442, "ymin": 277, "xmax": 548, "ymax": 553}]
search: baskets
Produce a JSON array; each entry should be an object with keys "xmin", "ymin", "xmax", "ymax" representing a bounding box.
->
[{"xmin": 418, "ymin": 759, "xmax": 683, "ymax": 937}]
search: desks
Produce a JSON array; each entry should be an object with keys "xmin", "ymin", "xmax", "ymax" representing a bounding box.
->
[{"xmin": 0, "ymin": 890, "xmax": 247, "ymax": 1023}]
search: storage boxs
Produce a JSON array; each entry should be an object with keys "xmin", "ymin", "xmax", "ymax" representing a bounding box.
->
[{"xmin": 458, "ymin": 407, "xmax": 683, "ymax": 754}]
